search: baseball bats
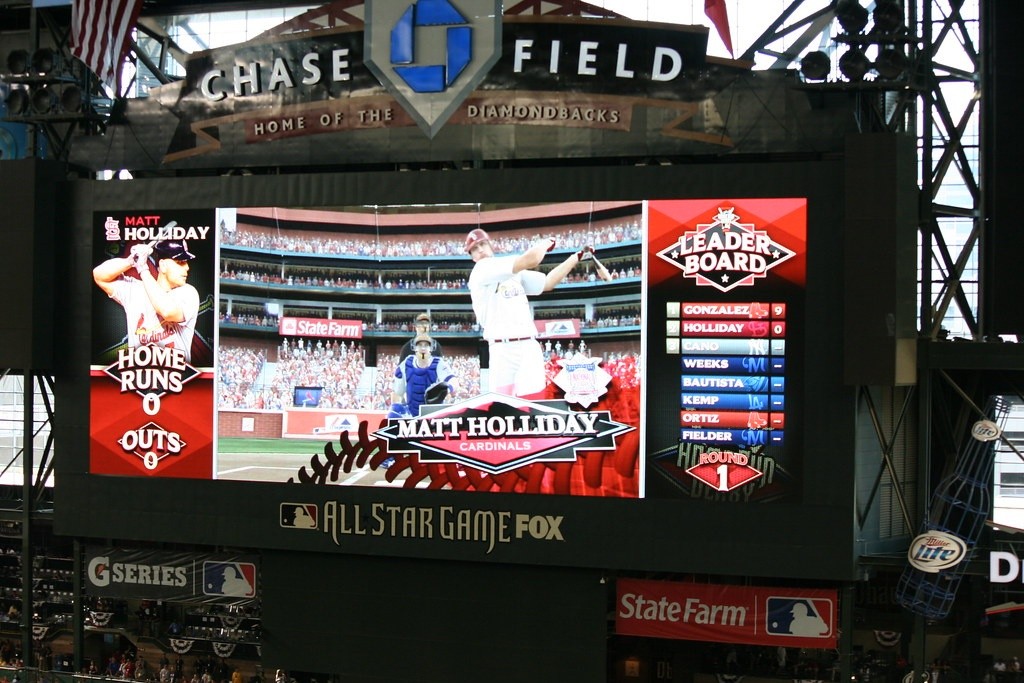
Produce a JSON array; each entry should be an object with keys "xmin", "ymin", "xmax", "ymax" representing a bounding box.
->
[
  {"xmin": 584, "ymin": 246, "xmax": 613, "ymax": 283},
  {"xmin": 132, "ymin": 219, "xmax": 177, "ymax": 262}
]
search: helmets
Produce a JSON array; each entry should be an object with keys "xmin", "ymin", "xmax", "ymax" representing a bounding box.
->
[
  {"xmin": 463, "ymin": 229, "xmax": 496, "ymax": 253},
  {"xmin": 415, "ymin": 314, "xmax": 430, "ymax": 324},
  {"xmin": 414, "ymin": 333, "xmax": 433, "ymax": 347}
]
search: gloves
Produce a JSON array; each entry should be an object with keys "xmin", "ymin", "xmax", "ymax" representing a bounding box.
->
[
  {"xmin": 576, "ymin": 246, "xmax": 596, "ymax": 262},
  {"xmin": 129, "ymin": 244, "xmax": 153, "ymax": 275},
  {"xmin": 546, "ymin": 236, "xmax": 556, "ymax": 252}
]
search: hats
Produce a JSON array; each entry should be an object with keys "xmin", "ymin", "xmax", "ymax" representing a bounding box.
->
[{"xmin": 155, "ymin": 238, "xmax": 195, "ymax": 261}]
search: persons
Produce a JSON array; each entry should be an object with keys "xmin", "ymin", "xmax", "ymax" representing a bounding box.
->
[
  {"xmin": 220, "ymin": 265, "xmax": 641, "ymax": 289},
  {"xmin": 219, "ymin": 310, "xmax": 641, "ymax": 331},
  {"xmin": 383, "ymin": 334, "xmax": 459, "ymax": 469},
  {"xmin": 219, "ymin": 218, "xmax": 641, "ymax": 258},
  {"xmin": 397, "ymin": 313, "xmax": 443, "ymax": 364},
  {"xmin": 92, "ymin": 235, "xmax": 200, "ymax": 365},
  {"xmin": 464, "ymin": 228, "xmax": 593, "ymax": 401},
  {"xmin": 217, "ymin": 338, "xmax": 589, "ymax": 415},
  {"xmin": 776, "ymin": 644, "xmax": 1024, "ymax": 683},
  {"xmin": 0, "ymin": 638, "xmax": 287, "ymax": 683}
]
